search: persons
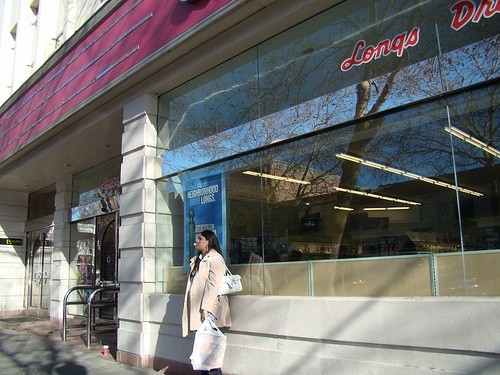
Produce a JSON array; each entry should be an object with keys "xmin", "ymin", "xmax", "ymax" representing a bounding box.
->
[
  {"xmin": 233, "ymin": 222, "xmax": 486, "ymax": 297},
  {"xmin": 181, "ymin": 230, "xmax": 232, "ymax": 375}
]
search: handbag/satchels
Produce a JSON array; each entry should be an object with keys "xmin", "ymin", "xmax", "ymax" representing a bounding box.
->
[
  {"xmin": 241, "ymin": 253, "xmax": 267, "ymax": 294},
  {"xmin": 210, "ymin": 256, "xmax": 242, "ymax": 295},
  {"xmin": 189, "ymin": 316, "xmax": 227, "ymax": 371}
]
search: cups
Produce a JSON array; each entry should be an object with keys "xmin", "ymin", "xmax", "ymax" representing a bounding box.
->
[{"xmin": 103, "ymin": 346, "xmax": 109, "ymax": 355}]
